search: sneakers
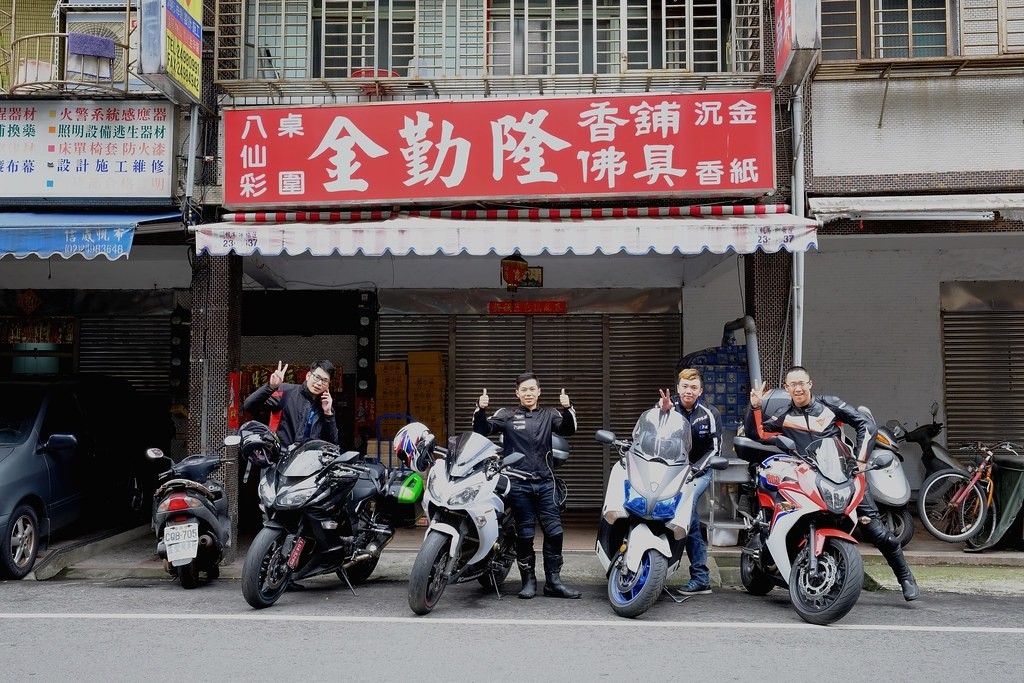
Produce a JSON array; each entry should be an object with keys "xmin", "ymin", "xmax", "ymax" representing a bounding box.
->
[{"xmin": 676, "ymin": 580, "xmax": 712, "ymax": 595}]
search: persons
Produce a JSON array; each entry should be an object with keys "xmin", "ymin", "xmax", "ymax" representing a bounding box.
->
[
  {"xmin": 746, "ymin": 366, "xmax": 920, "ymax": 601},
  {"xmin": 653, "ymin": 369, "xmax": 724, "ymax": 595},
  {"xmin": 472, "ymin": 373, "xmax": 582, "ymax": 598},
  {"xmin": 244, "ymin": 359, "xmax": 338, "ymax": 476}
]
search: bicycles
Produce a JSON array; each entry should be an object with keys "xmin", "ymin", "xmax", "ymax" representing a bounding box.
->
[{"xmin": 914, "ymin": 439, "xmax": 1024, "ymax": 544}]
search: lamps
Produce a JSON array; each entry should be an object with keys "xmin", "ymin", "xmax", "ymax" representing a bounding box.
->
[{"xmin": 850, "ymin": 210, "xmax": 1001, "ymax": 221}]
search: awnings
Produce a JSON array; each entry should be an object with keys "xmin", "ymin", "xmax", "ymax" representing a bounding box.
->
[
  {"xmin": 190, "ymin": 213, "xmax": 819, "ymax": 257},
  {"xmin": 0, "ymin": 209, "xmax": 183, "ymax": 261}
]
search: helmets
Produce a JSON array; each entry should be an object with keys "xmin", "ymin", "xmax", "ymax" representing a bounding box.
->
[
  {"xmin": 394, "ymin": 422, "xmax": 435, "ymax": 471},
  {"xmin": 238, "ymin": 420, "xmax": 281, "ymax": 468}
]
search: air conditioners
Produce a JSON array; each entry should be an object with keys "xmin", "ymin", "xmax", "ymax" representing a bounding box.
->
[{"xmin": 63, "ymin": 12, "xmax": 139, "ymax": 82}]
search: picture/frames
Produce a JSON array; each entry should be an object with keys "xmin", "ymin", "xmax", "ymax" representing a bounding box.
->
[{"xmin": 517, "ymin": 267, "xmax": 543, "ymax": 287}]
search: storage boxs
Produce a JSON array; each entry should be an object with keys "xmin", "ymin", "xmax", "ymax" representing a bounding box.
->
[
  {"xmin": 367, "ymin": 437, "xmax": 402, "ymax": 466},
  {"xmin": 500, "ymin": 433, "xmax": 569, "ymax": 470},
  {"xmin": 374, "ymin": 362, "xmax": 407, "ymax": 438},
  {"xmin": 383, "ymin": 470, "xmax": 424, "ymax": 528},
  {"xmin": 407, "ymin": 352, "xmax": 446, "ymax": 460},
  {"xmin": 732, "ymin": 388, "xmax": 793, "ymax": 463}
]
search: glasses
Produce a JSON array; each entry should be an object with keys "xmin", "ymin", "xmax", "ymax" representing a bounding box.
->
[
  {"xmin": 311, "ymin": 372, "xmax": 331, "ymax": 385},
  {"xmin": 786, "ymin": 381, "xmax": 808, "ymax": 388}
]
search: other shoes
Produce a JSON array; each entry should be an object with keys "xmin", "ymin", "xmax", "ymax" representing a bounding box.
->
[{"xmin": 286, "ymin": 581, "xmax": 305, "ymax": 591}]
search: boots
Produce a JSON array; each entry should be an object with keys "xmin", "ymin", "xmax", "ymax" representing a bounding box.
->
[
  {"xmin": 543, "ymin": 551, "xmax": 582, "ymax": 599},
  {"xmin": 517, "ymin": 552, "xmax": 537, "ymax": 598},
  {"xmin": 884, "ymin": 547, "xmax": 921, "ymax": 601}
]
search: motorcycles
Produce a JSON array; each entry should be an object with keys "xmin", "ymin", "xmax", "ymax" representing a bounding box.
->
[
  {"xmin": 407, "ymin": 429, "xmax": 572, "ymax": 614},
  {"xmin": 595, "ymin": 408, "xmax": 729, "ymax": 617},
  {"xmin": 241, "ymin": 437, "xmax": 427, "ymax": 608},
  {"xmin": 732, "ymin": 432, "xmax": 873, "ymax": 626}
]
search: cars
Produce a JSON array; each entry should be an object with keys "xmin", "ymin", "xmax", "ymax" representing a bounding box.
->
[{"xmin": 0, "ymin": 380, "xmax": 150, "ymax": 580}]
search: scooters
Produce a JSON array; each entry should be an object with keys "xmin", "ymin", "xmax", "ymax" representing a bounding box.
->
[
  {"xmin": 845, "ymin": 406, "xmax": 916, "ymax": 551},
  {"xmin": 885, "ymin": 401, "xmax": 972, "ymax": 522},
  {"xmin": 144, "ymin": 435, "xmax": 243, "ymax": 588}
]
